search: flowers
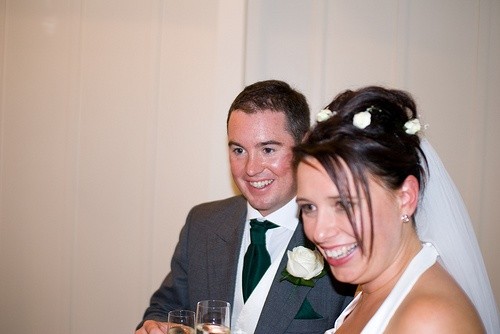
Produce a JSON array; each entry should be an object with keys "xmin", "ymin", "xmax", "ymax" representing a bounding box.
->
[
  {"xmin": 352, "ymin": 111, "xmax": 372, "ymax": 130},
  {"xmin": 403, "ymin": 118, "xmax": 421, "ymax": 135},
  {"xmin": 278, "ymin": 246, "xmax": 328, "ymax": 288},
  {"xmin": 315, "ymin": 109, "xmax": 336, "ymax": 123}
]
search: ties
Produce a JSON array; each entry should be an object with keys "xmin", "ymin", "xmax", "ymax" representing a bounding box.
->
[{"xmin": 242, "ymin": 219, "xmax": 281, "ymax": 304}]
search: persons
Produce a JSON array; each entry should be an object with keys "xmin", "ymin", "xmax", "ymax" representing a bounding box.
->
[{"xmin": 135, "ymin": 79, "xmax": 491, "ymax": 334}]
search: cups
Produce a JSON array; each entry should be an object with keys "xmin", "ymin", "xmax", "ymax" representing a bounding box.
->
[
  {"xmin": 196, "ymin": 300, "xmax": 230, "ymax": 334},
  {"xmin": 168, "ymin": 310, "xmax": 195, "ymax": 334}
]
